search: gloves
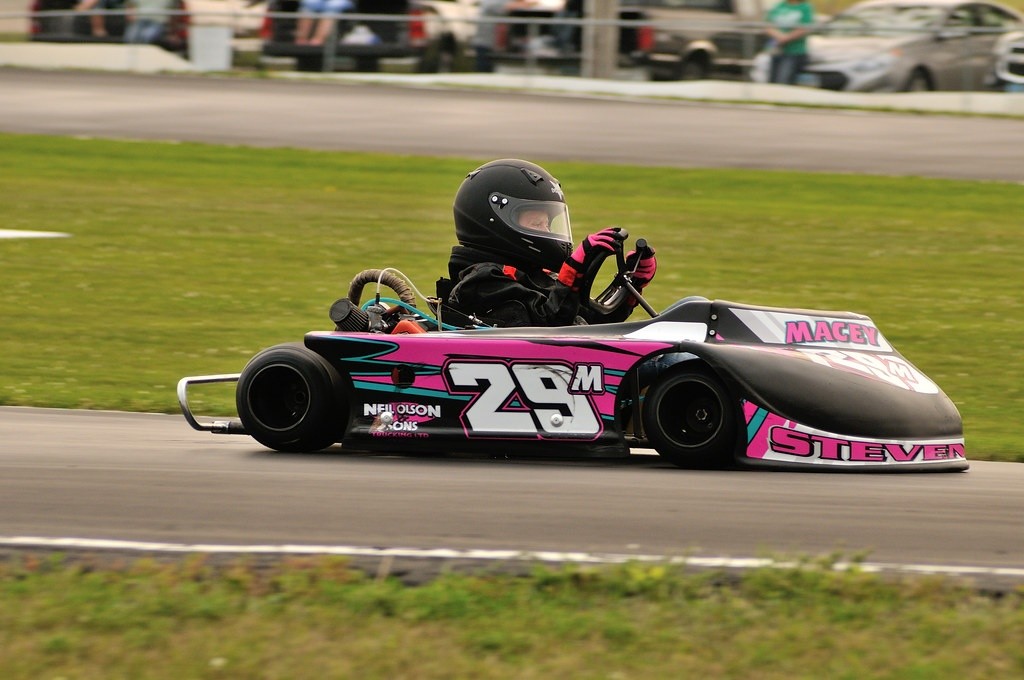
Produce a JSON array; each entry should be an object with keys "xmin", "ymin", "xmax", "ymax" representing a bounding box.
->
[
  {"xmin": 555, "ymin": 227, "xmax": 630, "ymax": 292},
  {"xmin": 626, "ymin": 248, "xmax": 658, "ymax": 306}
]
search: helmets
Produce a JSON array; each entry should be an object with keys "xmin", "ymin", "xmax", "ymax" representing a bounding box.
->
[{"xmin": 453, "ymin": 156, "xmax": 574, "ymax": 275}]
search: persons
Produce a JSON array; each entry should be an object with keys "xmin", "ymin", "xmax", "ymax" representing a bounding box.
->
[
  {"xmin": 53, "ymin": 0, "xmax": 108, "ymax": 41},
  {"xmin": 762, "ymin": 0, "xmax": 816, "ymax": 88},
  {"xmin": 283, "ymin": 0, "xmax": 362, "ymax": 51},
  {"xmin": 437, "ymin": 156, "xmax": 659, "ymax": 333},
  {"xmin": 120, "ymin": 1, "xmax": 181, "ymax": 47}
]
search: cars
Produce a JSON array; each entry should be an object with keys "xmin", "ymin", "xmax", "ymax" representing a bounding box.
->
[{"xmin": 22, "ymin": 0, "xmax": 1024, "ymax": 97}]
